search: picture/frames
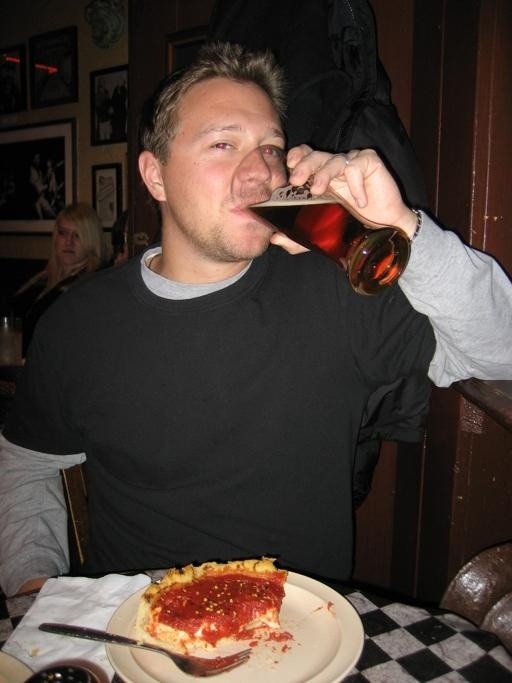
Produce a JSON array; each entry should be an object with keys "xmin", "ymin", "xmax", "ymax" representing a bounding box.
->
[{"xmin": 2, "ymin": 24, "xmax": 129, "ymax": 235}]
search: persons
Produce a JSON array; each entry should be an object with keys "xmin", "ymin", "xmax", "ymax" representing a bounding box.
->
[
  {"xmin": 0, "ymin": 39, "xmax": 511, "ymax": 599},
  {"xmin": 1, "ymin": 199, "xmax": 106, "ymax": 368},
  {"xmin": 108, "ymin": 70, "xmax": 128, "ymax": 141},
  {"xmin": 43, "ymin": 154, "xmax": 62, "ymax": 207},
  {"xmin": 26, "ymin": 151, "xmax": 59, "ymax": 220},
  {"xmin": 94, "ymin": 74, "xmax": 114, "ymax": 141}
]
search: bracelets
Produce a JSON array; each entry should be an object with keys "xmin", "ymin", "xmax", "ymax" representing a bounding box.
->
[{"xmin": 409, "ymin": 209, "xmax": 423, "ymax": 243}]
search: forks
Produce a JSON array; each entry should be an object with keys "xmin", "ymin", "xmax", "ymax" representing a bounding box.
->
[{"xmin": 38, "ymin": 621, "xmax": 252, "ymax": 679}]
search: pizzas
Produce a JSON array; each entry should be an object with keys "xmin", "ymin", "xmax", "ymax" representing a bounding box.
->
[{"xmin": 136, "ymin": 555, "xmax": 295, "ymax": 660}]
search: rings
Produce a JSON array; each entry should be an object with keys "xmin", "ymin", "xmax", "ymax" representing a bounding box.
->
[{"xmin": 331, "ymin": 151, "xmax": 352, "ymax": 175}]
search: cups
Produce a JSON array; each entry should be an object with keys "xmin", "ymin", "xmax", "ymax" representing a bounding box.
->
[
  {"xmin": 227, "ymin": 143, "xmax": 413, "ymax": 299},
  {"xmin": 1, "ymin": 318, "xmax": 26, "ymax": 367}
]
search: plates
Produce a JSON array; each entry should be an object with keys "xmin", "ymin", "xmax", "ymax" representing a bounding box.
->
[{"xmin": 104, "ymin": 567, "xmax": 366, "ymax": 683}]
